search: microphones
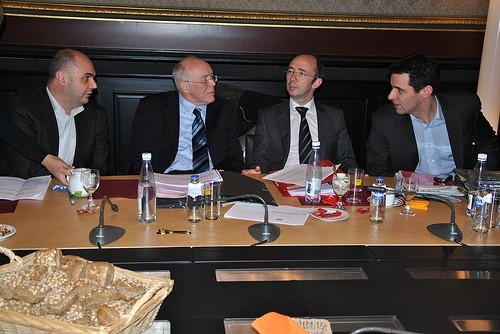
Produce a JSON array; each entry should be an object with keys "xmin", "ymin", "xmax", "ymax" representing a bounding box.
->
[
  {"xmin": 212, "ymin": 194, "xmax": 280, "ymax": 242},
  {"xmin": 402, "ymin": 193, "xmax": 464, "ymax": 243},
  {"xmin": 89, "ymin": 195, "xmax": 125, "ymax": 246}
]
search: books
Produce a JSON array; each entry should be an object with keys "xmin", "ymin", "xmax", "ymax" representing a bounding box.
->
[
  {"xmin": 0, "ymin": 175, "xmax": 52, "ymax": 201},
  {"xmin": 395, "ymin": 170, "xmax": 465, "ymax": 196}
]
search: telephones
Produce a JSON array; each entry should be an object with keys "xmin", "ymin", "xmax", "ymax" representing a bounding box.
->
[{"xmin": 433, "ymin": 173, "xmax": 453, "ymax": 183}]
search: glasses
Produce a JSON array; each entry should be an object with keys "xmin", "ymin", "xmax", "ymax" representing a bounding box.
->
[
  {"xmin": 179, "ymin": 75, "xmax": 218, "ymax": 84},
  {"xmin": 283, "ymin": 69, "xmax": 318, "ymax": 79}
]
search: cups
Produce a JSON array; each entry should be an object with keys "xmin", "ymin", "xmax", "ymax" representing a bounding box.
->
[
  {"xmin": 472, "ymin": 184, "xmax": 492, "ymax": 233},
  {"xmin": 386, "ymin": 187, "xmax": 395, "ymax": 207},
  {"xmin": 346, "ymin": 168, "xmax": 364, "ymax": 203},
  {"xmin": 67, "ymin": 168, "xmax": 91, "ymax": 197},
  {"xmin": 490, "ymin": 189, "xmax": 500, "ymax": 230},
  {"xmin": 204, "ymin": 181, "xmax": 221, "ymax": 220}
]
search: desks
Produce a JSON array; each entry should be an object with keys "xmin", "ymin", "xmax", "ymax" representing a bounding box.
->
[{"xmin": 0, "ymin": 174, "xmax": 500, "ymax": 262}]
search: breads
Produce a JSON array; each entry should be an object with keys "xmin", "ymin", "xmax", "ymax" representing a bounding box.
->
[{"xmin": 0, "ymin": 248, "xmax": 149, "ymax": 326}]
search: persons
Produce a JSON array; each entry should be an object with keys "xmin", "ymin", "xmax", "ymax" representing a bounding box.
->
[
  {"xmin": 365, "ymin": 56, "xmax": 500, "ymax": 177},
  {"xmin": 128, "ymin": 57, "xmax": 244, "ymax": 175},
  {"xmin": 242, "ymin": 53, "xmax": 369, "ymax": 176},
  {"xmin": 0, "ymin": 49, "xmax": 109, "ymax": 187}
]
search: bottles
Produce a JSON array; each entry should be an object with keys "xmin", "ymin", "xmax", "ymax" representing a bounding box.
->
[
  {"xmin": 187, "ymin": 175, "xmax": 202, "ymax": 223},
  {"xmin": 304, "ymin": 141, "xmax": 322, "ymax": 204},
  {"xmin": 466, "ymin": 153, "xmax": 489, "ymax": 217},
  {"xmin": 370, "ymin": 176, "xmax": 386, "ymax": 223},
  {"xmin": 137, "ymin": 153, "xmax": 157, "ymax": 222}
]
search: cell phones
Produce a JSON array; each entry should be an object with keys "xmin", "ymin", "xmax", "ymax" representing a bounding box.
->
[{"xmin": 434, "ymin": 173, "xmax": 453, "ymax": 181}]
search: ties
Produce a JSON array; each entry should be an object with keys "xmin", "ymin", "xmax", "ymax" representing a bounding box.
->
[
  {"xmin": 191, "ymin": 108, "xmax": 210, "ymax": 174},
  {"xmin": 295, "ymin": 107, "xmax": 315, "ymax": 164}
]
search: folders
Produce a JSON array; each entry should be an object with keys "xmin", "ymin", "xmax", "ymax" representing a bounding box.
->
[
  {"xmin": 154, "ymin": 169, "xmax": 280, "ymax": 208},
  {"xmin": 268, "ymin": 159, "xmax": 348, "ymax": 197}
]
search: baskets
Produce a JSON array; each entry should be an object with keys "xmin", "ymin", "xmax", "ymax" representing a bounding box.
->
[
  {"xmin": 0, "ymin": 251, "xmax": 174, "ymax": 334},
  {"xmin": 290, "ymin": 317, "xmax": 332, "ymax": 334}
]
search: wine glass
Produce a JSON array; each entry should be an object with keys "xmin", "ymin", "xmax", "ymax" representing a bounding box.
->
[
  {"xmin": 81, "ymin": 169, "xmax": 100, "ymax": 209},
  {"xmin": 333, "ymin": 174, "xmax": 350, "ymax": 210},
  {"xmin": 399, "ymin": 177, "xmax": 418, "ymax": 216}
]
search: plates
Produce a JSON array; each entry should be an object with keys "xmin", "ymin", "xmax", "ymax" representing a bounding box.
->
[
  {"xmin": 367, "ymin": 196, "xmax": 403, "ymax": 207},
  {"xmin": 0, "ymin": 224, "xmax": 16, "ymax": 238}
]
